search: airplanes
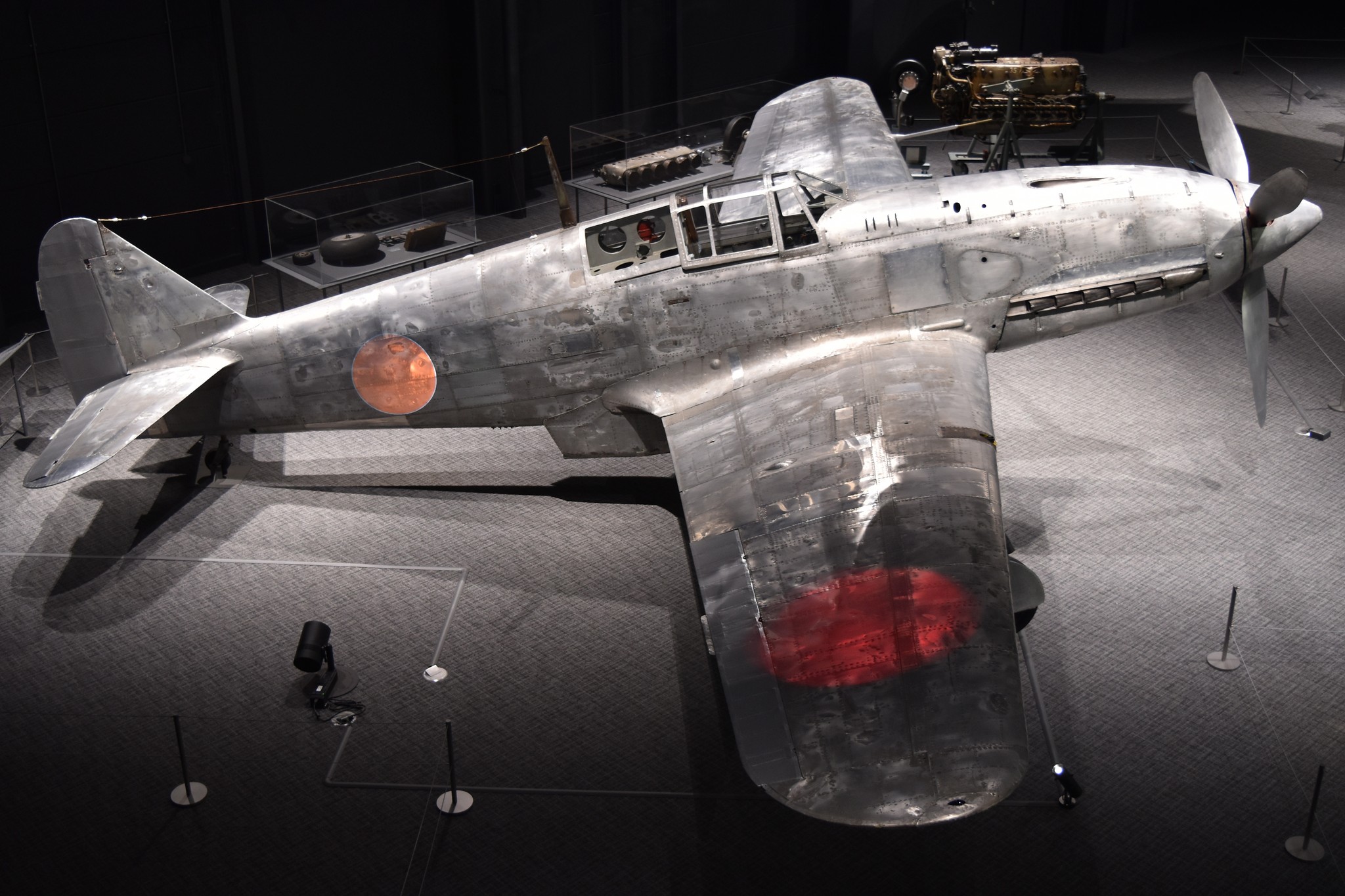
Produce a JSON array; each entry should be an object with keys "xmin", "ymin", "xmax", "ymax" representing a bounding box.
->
[{"xmin": 20, "ymin": 77, "xmax": 1326, "ymax": 827}]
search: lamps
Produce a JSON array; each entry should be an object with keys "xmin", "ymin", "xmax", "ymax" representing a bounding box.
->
[
  {"xmin": 1051, "ymin": 763, "xmax": 1084, "ymax": 806},
  {"xmin": 292, "ymin": 621, "xmax": 342, "ymax": 714}
]
